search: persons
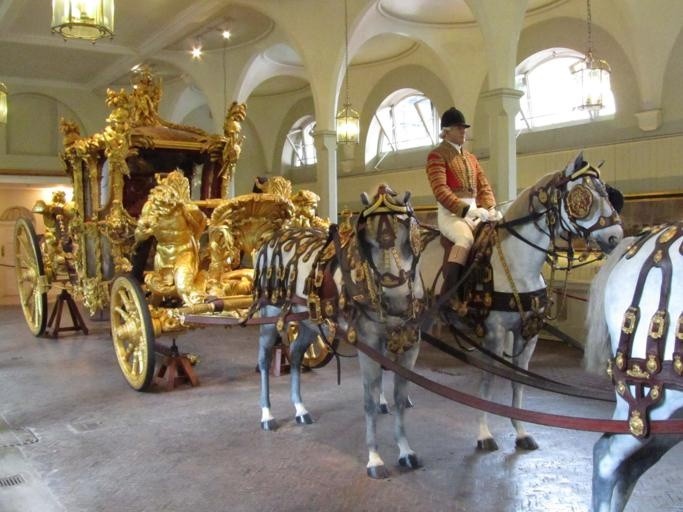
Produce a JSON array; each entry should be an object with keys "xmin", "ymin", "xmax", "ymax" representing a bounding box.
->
[
  {"xmin": 415, "ymin": 106, "xmax": 505, "ymax": 317},
  {"xmin": 130, "ymin": 185, "xmax": 206, "ymax": 298},
  {"xmin": 291, "ymin": 189, "xmax": 321, "ymax": 229}
]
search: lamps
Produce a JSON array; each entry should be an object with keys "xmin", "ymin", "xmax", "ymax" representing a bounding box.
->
[
  {"xmin": 569, "ymin": 0, "xmax": 612, "ymax": 122},
  {"xmin": 47, "ymin": 0, "xmax": 116, "ymax": 43},
  {"xmin": 0, "ymin": 80, "xmax": 10, "ymax": 129},
  {"xmin": 332, "ymin": 1, "xmax": 364, "ymax": 146}
]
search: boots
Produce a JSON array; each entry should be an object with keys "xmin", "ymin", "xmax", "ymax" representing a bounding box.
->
[{"xmin": 440, "ymin": 261, "xmax": 478, "ymax": 315}]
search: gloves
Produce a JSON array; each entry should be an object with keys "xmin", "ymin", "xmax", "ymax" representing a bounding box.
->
[{"xmin": 468, "ymin": 207, "xmax": 497, "ymax": 222}]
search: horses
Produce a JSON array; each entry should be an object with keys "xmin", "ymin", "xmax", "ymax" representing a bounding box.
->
[
  {"xmin": 579, "ymin": 220, "xmax": 683, "ymax": 512},
  {"xmin": 377, "ymin": 152, "xmax": 625, "ymax": 454},
  {"xmin": 247, "ymin": 181, "xmax": 428, "ymax": 480}
]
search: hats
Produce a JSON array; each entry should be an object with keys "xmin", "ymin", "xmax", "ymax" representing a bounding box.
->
[{"xmin": 441, "ymin": 107, "xmax": 471, "ymax": 130}]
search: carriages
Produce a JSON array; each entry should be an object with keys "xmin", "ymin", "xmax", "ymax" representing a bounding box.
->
[{"xmin": 13, "ymin": 55, "xmax": 682, "ymax": 512}]
